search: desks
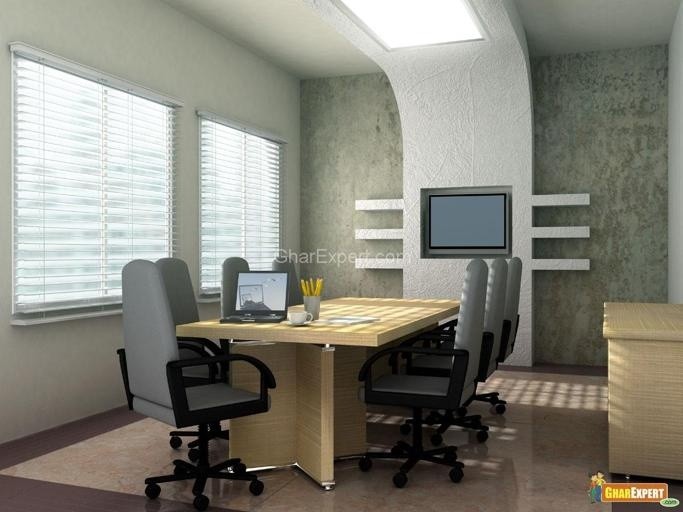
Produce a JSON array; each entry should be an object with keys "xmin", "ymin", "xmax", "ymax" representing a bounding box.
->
[
  {"xmin": 603, "ymin": 299, "xmax": 683, "ymax": 483},
  {"xmin": 175, "ymin": 297, "xmax": 462, "ymax": 491}
]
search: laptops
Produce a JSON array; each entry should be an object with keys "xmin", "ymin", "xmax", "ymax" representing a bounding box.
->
[{"xmin": 219, "ymin": 270, "xmax": 290, "ymax": 323}]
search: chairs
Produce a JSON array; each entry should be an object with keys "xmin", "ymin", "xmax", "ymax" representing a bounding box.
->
[
  {"xmin": 219, "ymin": 256, "xmax": 249, "ymax": 321},
  {"xmin": 426, "ymin": 257, "xmax": 523, "ymax": 416},
  {"xmin": 155, "ymin": 257, "xmax": 229, "ymax": 462},
  {"xmin": 115, "ymin": 259, "xmax": 276, "ymax": 511},
  {"xmin": 396, "ymin": 259, "xmax": 508, "ymax": 445},
  {"xmin": 353, "ymin": 259, "xmax": 488, "ymax": 489}
]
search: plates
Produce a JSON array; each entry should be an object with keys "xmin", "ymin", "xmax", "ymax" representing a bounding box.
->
[{"xmin": 281, "ymin": 320, "xmax": 312, "ymax": 326}]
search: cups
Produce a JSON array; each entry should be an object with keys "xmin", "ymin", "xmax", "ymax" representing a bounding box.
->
[
  {"xmin": 304, "ymin": 295, "xmax": 321, "ymax": 320},
  {"xmin": 287, "ymin": 311, "xmax": 314, "ymax": 323}
]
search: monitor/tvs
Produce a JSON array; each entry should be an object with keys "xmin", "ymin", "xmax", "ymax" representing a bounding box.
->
[{"xmin": 425, "ymin": 190, "xmax": 510, "ymax": 256}]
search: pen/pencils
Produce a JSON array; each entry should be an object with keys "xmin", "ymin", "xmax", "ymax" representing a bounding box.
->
[
  {"xmin": 314, "ymin": 277, "xmax": 324, "ymax": 296},
  {"xmin": 310, "ymin": 278, "xmax": 314, "ymax": 295},
  {"xmin": 300, "ymin": 276, "xmax": 310, "ymax": 296}
]
